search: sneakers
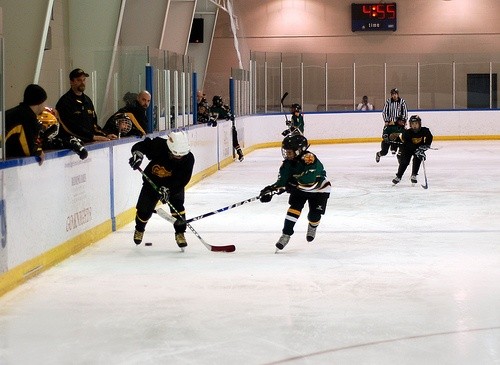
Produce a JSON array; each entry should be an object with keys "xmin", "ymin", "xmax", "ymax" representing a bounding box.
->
[
  {"xmin": 274, "ymin": 234, "xmax": 291, "ymax": 253},
  {"xmin": 238, "ymin": 150, "xmax": 245, "ymax": 162},
  {"xmin": 133, "ymin": 229, "xmax": 144, "ymax": 246},
  {"xmin": 175, "ymin": 232, "xmax": 187, "ymax": 252},
  {"xmin": 392, "ymin": 177, "xmax": 400, "ymax": 184},
  {"xmin": 306, "ymin": 223, "xmax": 319, "ymax": 242},
  {"xmin": 410, "ymin": 174, "xmax": 417, "ymax": 183}
]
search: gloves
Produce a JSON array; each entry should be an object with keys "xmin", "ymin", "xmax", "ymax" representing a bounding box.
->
[
  {"xmin": 397, "ymin": 150, "xmax": 402, "ymax": 164},
  {"xmin": 415, "ymin": 145, "xmax": 429, "ymax": 160},
  {"xmin": 67, "ymin": 136, "xmax": 88, "ymax": 160},
  {"xmin": 207, "ymin": 118, "xmax": 217, "ymax": 127},
  {"xmin": 260, "ymin": 185, "xmax": 274, "ymax": 203},
  {"xmin": 284, "ymin": 177, "xmax": 298, "ymax": 193},
  {"xmin": 281, "ymin": 130, "xmax": 290, "ymax": 136},
  {"xmin": 159, "ymin": 185, "xmax": 170, "ymax": 205},
  {"xmin": 129, "ymin": 151, "xmax": 144, "ymax": 170},
  {"xmin": 286, "ymin": 121, "xmax": 291, "ymax": 125},
  {"xmin": 382, "ymin": 134, "xmax": 389, "ymax": 143}
]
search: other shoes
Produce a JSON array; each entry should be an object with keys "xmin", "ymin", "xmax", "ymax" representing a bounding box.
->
[{"xmin": 376, "ymin": 152, "xmax": 380, "ymax": 162}]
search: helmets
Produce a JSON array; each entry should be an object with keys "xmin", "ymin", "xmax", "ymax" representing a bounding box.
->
[
  {"xmin": 409, "ymin": 115, "xmax": 421, "ymax": 133},
  {"xmin": 282, "ymin": 134, "xmax": 308, "ymax": 156},
  {"xmin": 212, "ymin": 95, "xmax": 223, "ymax": 106},
  {"xmin": 114, "ymin": 113, "xmax": 133, "ymax": 135},
  {"xmin": 292, "ymin": 104, "xmax": 301, "ymax": 111},
  {"xmin": 166, "ymin": 130, "xmax": 191, "ymax": 156},
  {"xmin": 37, "ymin": 111, "xmax": 57, "ymax": 129}
]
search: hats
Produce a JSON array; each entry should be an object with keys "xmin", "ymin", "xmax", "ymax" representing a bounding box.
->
[
  {"xmin": 69, "ymin": 68, "xmax": 89, "ymax": 77},
  {"xmin": 23, "ymin": 84, "xmax": 47, "ymax": 105},
  {"xmin": 391, "ymin": 88, "xmax": 399, "ymax": 93}
]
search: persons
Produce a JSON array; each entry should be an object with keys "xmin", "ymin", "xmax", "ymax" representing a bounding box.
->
[
  {"xmin": 129, "ymin": 130, "xmax": 194, "ymax": 248},
  {"xmin": 55, "ymin": 68, "xmax": 118, "ymax": 144},
  {"xmin": 376, "ymin": 116, "xmax": 407, "ymax": 162},
  {"xmin": 382, "ymin": 88, "xmax": 408, "ymax": 154},
  {"xmin": 392, "ymin": 115, "xmax": 433, "ymax": 184},
  {"xmin": 259, "ymin": 134, "xmax": 331, "ymax": 250},
  {"xmin": 282, "ymin": 104, "xmax": 304, "ymax": 136},
  {"xmin": 357, "ymin": 96, "xmax": 374, "ymax": 111},
  {"xmin": 103, "ymin": 90, "xmax": 153, "ymax": 137},
  {"xmin": 5, "ymin": 83, "xmax": 87, "ymax": 166},
  {"xmin": 197, "ymin": 89, "xmax": 243, "ymax": 160}
]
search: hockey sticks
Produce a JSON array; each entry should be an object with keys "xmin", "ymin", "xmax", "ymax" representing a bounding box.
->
[
  {"xmin": 421, "ymin": 156, "xmax": 428, "ymax": 189},
  {"xmin": 138, "ymin": 166, "xmax": 236, "ymax": 253},
  {"xmin": 389, "ymin": 140, "xmax": 441, "ymax": 151},
  {"xmin": 281, "ymin": 92, "xmax": 289, "ymax": 121},
  {"xmin": 157, "ymin": 185, "xmax": 286, "ymax": 226}
]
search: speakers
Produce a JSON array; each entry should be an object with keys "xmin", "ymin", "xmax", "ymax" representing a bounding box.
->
[{"xmin": 190, "ymin": 18, "xmax": 203, "ymax": 43}]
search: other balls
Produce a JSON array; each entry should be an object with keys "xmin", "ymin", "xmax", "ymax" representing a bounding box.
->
[{"xmin": 145, "ymin": 243, "xmax": 152, "ymax": 246}]
